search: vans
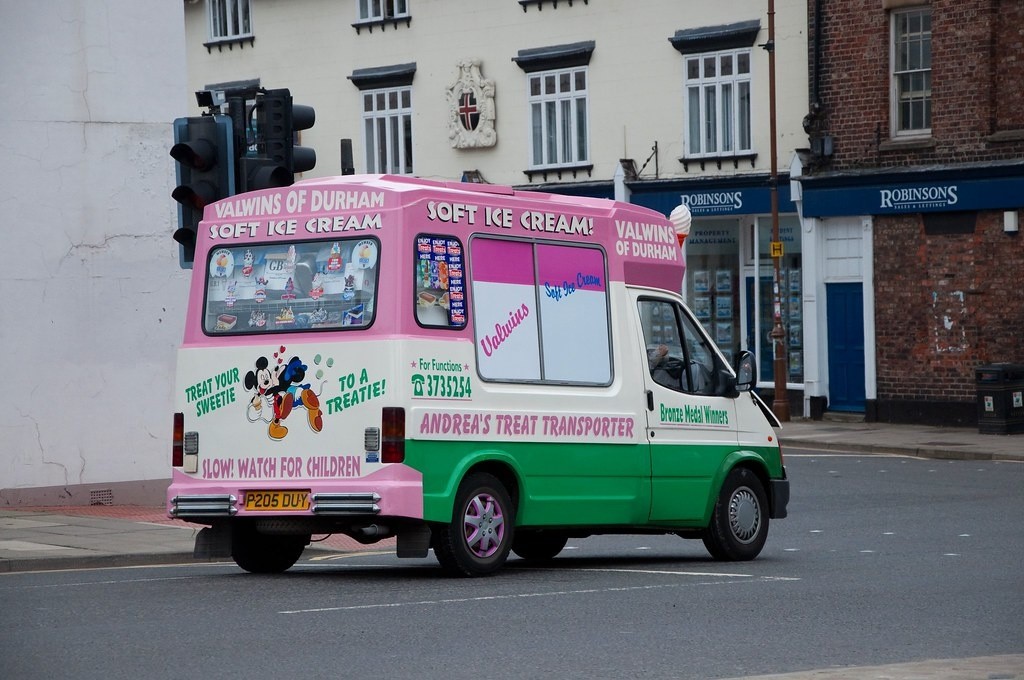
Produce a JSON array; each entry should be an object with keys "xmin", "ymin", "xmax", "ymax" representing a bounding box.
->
[{"xmin": 164, "ymin": 171, "xmax": 794, "ymax": 579}]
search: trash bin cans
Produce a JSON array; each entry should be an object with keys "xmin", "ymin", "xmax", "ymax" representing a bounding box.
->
[{"xmin": 975, "ymin": 363, "xmax": 1024, "ymax": 435}]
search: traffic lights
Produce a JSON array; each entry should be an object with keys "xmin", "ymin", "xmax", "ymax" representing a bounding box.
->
[
  {"xmin": 168, "ymin": 114, "xmax": 237, "ymax": 269},
  {"xmin": 241, "ymin": 157, "xmax": 295, "ymax": 196},
  {"xmin": 255, "ymin": 87, "xmax": 317, "ymax": 174}
]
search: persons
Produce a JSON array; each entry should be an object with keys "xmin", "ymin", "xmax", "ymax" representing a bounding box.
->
[
  {"xmin": 740, "ymin": 363, "xmax": 752, "ymax": 383},
  {"xmin": 649, "ymin": 344, "xmax": 669, "ymax": 371}
]
